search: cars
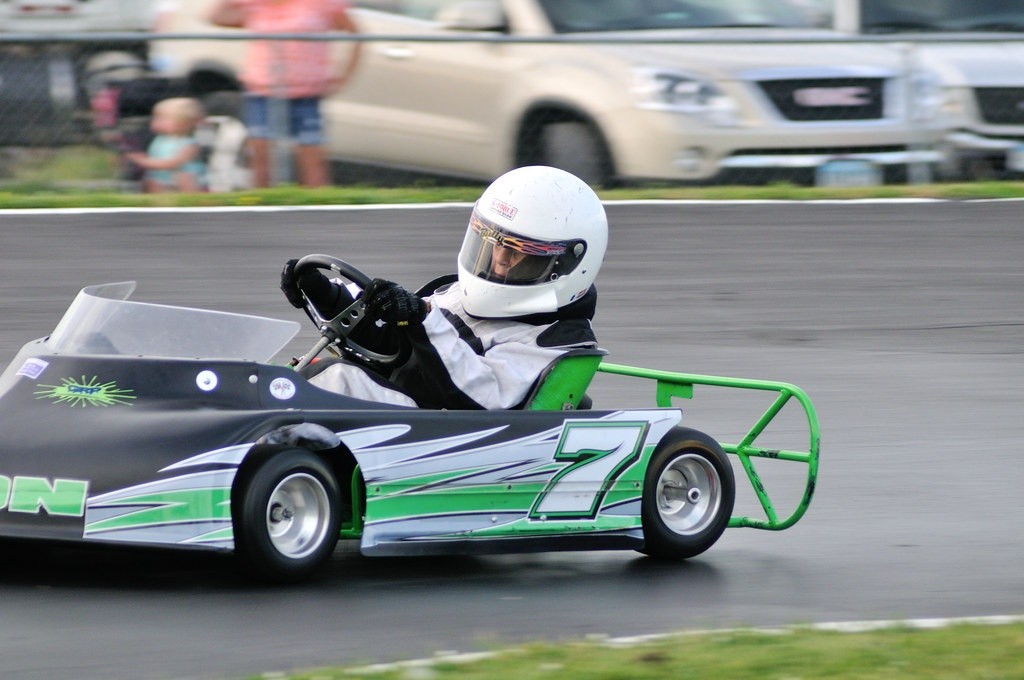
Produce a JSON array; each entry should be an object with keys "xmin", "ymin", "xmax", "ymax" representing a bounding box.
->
[
  {"xmin": 830, "ymin": 1, "xmax": 1024, "ymax": 179},
  {"xmin": 145, "ymin": 1, "xmax": 960, "ymax": 185}
]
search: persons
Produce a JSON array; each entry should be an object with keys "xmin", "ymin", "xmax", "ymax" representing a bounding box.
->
[
  {"xmin": 281, "ymin": 166, "xmax": 608, "ymax": 410},
  {"xmin": 208, "ymin": 0, "xmax": 360, "ymax": 189},
  {"xmin": 125, "ymin": 97, "xmax": 208, "ymax": 195}
]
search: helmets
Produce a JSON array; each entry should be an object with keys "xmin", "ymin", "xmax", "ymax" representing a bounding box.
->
[{"xmin": 458, "ymin": 164, "xmax": 608, "ymax": 318}]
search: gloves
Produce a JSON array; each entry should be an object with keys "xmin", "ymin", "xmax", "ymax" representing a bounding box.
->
[
  {"xmin": 280, "ymin": 258, "xmax": 339, "ymax": 313},
  {"xmin": 360, "ymin": 279, "xmax": 426, "ymax": 326}
]
